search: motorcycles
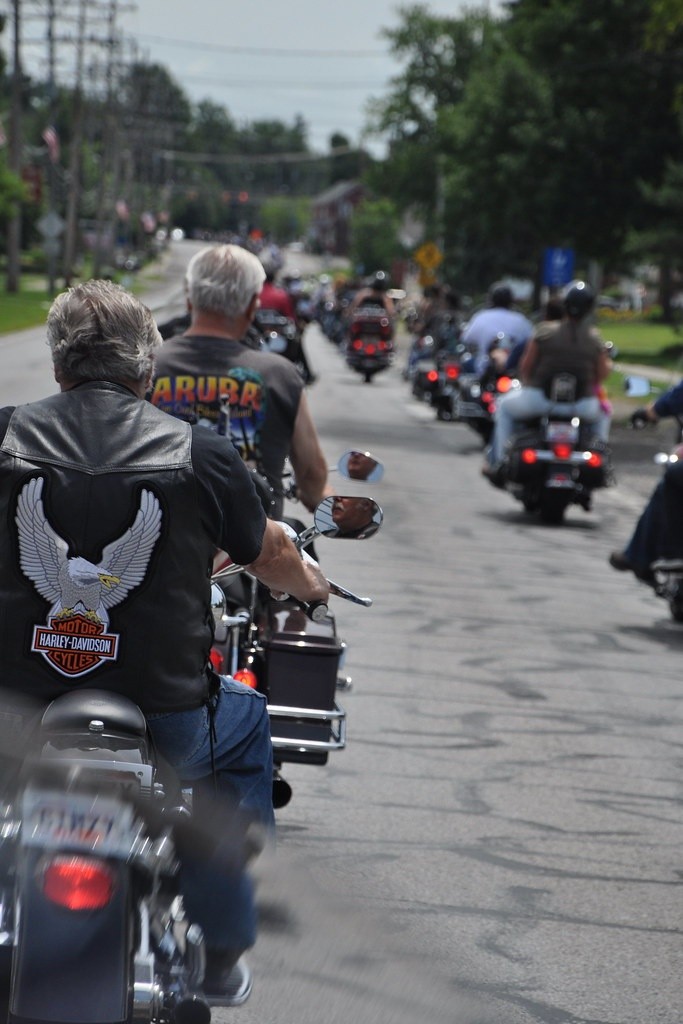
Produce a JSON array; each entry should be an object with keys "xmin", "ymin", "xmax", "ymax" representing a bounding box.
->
[{"xmin": 1, "ymin": 225, "xmax": 680, "ymax": 1023}]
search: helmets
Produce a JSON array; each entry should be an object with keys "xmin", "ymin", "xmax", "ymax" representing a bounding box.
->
[
  {"xmin": 371, "ymin": 270, "xmax": 390, "ymax": 290},
  {"xmin": 492, "ymin": 284, "xmax": 512, "ymax": 303},
  {"xmin": 260, "ymin": 244, "xmax": 283, "ymax": 272},
  {"xmin": 563, "ymin": 279, "xmax": 596, "ymax": 308}
]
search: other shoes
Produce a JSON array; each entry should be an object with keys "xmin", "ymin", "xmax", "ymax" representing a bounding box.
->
[
  {"xmin": 610, "ymin": 552, "xmax": 633, "ymax": 570},
  {"xmin": 482, "ymin": 464, "xmax": 505, "ymax": 487}
]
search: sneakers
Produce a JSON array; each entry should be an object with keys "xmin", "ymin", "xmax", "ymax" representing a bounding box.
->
[{"xmin": 207, "ymin": 955, "xmax": 254, "ymax": 1006}]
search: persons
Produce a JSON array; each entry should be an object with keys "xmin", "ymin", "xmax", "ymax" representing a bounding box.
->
[
  {"xmin": 146, "ymin": 243, "xmax": 328, "ymax": 802},
  {"xmin": 629, "ymin": 379, "xmax": 683, "ymax": 591},
  {"xmin": 0, "ymin": 282, "xmax": 332, "ymax": 975},
  {"xmin": 234, "ymin": 229, "xmax": 617, "ymax": 542}
]
